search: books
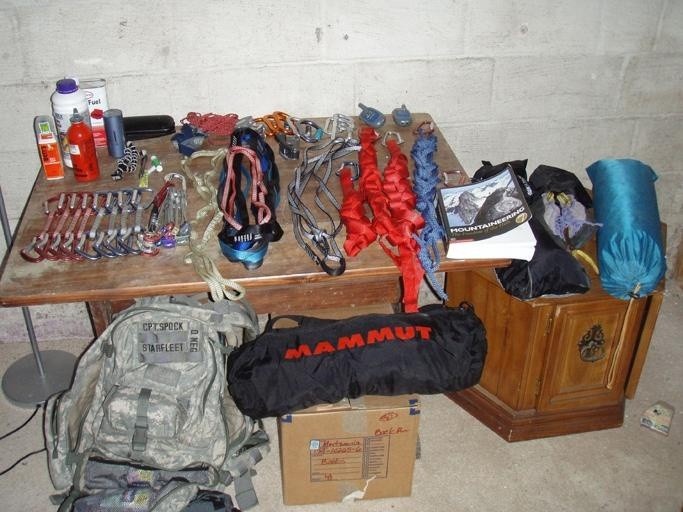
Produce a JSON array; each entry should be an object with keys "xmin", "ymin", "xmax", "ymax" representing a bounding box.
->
[{"xmin": 435, "ymin": 162, "xmax": 538, "ymax": 265}]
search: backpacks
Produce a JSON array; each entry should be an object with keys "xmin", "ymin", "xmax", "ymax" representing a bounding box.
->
[
  {"xmin": 41, "ymin": 289, "xmax": 271, "ymax": 511},
  {"xmin": 223, "ymin": 300, "xmax": 487, "ymax": 421}
]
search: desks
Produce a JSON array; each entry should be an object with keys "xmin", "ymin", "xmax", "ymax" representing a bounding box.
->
[{"xmin": 1, "ymin": 114, "xmax": 514, "ymax": 340}]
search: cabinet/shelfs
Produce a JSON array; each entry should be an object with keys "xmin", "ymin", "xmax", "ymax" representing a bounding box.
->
[{"xmin": 440, "ymin": 189, "xmax": 668, "ymax": 443}]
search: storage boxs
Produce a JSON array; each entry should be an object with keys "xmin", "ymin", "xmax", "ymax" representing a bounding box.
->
[{"xmin": 268, "ymin": 300, "xmax": 422, "ymax": 505}]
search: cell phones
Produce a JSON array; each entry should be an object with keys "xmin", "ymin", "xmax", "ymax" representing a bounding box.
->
[
  {"xmin": 358, "ymin": 102, "xmax": 386, "ymax": 129},
  {"xmin": 392, "ymin": 104, "xmax": 412, "ymax": 126}
]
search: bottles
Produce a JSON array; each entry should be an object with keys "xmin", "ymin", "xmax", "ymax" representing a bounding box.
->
[
  {"xmin": 65, "ymin": 109, "xmax": 97, "ymax": 181},
  {"xmin": 33, "ymin": 118, "xmax": 63, "ymax": 184},
  {"xmin": 77, "ymin": 79, "xmax": 108, "ymax": 149},
  {"xmin": 106, "ymin": 109, "xmax": 130, "ymax": 158},
  {"xmin": 49, "ymin": 80, "xmax": 96, "ymax": 173}
]
